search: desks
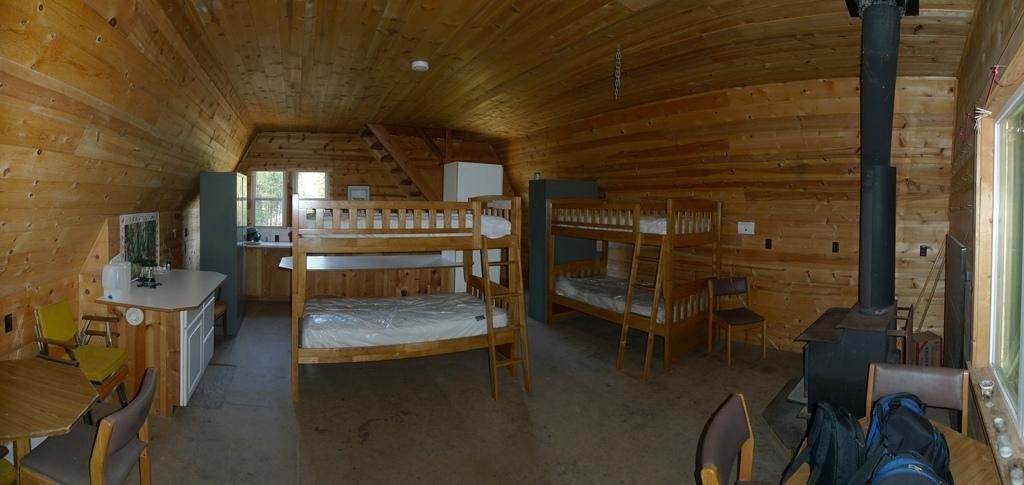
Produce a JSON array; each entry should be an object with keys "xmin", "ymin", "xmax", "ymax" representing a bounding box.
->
[
  {"xmin": 782, "ymin": 410, "xmax": 1003, "ymax": 485},
  {"xmin": 0, "ymin": 358, "xmax": 99, "ymax": 467}
]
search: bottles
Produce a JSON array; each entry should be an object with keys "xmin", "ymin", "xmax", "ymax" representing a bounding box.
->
[
  {"xmin": 534, "ymin": 172, "xmax": 540, "ymax": 180},
  {"xmin": 162, "ymin": 261, "xmax": 173, "ymax": 272}
]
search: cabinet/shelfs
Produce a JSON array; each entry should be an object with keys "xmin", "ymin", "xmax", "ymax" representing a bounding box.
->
[
  {"xmin": 528, "ymin": 181, "xmax": 599, "ymax": 321},
  {"xmin": 199, "ymin": 170, "xmax": 249, "ymax": 336},
  {"xmin": 92, "ymin": 272, "xmax": 228, "ymax": 417}
]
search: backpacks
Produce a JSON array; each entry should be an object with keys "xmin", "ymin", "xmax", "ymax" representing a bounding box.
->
[{"xmin": 779, "ymin": 402, "xmax": 867, "ymax": 485}]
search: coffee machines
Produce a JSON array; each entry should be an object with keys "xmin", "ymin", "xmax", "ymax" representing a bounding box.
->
[{"xmin": 135, "ymin": 261, "xmax": 160, "ymax": 288}]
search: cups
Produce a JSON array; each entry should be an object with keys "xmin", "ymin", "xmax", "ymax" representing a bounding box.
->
[
  {"xmin": 979, "ymin": 379, "xmax": 996, "ymax": 408},
  {"xmin": 274, "ymin": 234, "xmax": 279, "ymax": 242},
  {"xmin": 992, "ymin": 416, "xmax": 1023, "ymax": 485}
]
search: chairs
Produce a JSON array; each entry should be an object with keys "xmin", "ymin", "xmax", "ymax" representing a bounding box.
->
[
  {"xmin": 16, "ymin": 368, "xmax": 157, "ymax": 483},
  {"xmin": 698, "ymin": 391, "xmax": 779, "ymax": 484},
  {"xmin": 34, "ymin": 300, "xmax": 128, "ymax": 427},
  {"xmin": 709, "ymin": 273, "xmax": 768, "ymax": 367},
  {"xmin": 210, "ymin": 286, "xmax": 226, "ymax": 344},
  {"xmin": 864, "ymin": 364, "xmax": 969, "ymax": 440}
]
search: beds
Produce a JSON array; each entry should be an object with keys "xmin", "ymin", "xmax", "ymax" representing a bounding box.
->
[
  {"xmin": 546, "ymin": 198, "xmax": 721, "ymax": 380},
  {"xmin": 291, "ymin": 194, "xmax": 525, "ymax": 404}
]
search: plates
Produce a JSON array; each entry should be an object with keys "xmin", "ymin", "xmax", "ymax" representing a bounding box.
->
[{"xmin": 245, "ymin": 242, "xmax": 261, "ymax": 245}]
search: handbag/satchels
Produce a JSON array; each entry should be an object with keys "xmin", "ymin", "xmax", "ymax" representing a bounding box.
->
[{"xmin": 851, "ymin": 392, "xmax": 955, "ymax": 484}]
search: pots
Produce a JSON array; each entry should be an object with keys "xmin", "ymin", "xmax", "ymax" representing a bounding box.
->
[{"xmin": 247, "ymin": 228, "xmax": 262, "ymax": 242}]
